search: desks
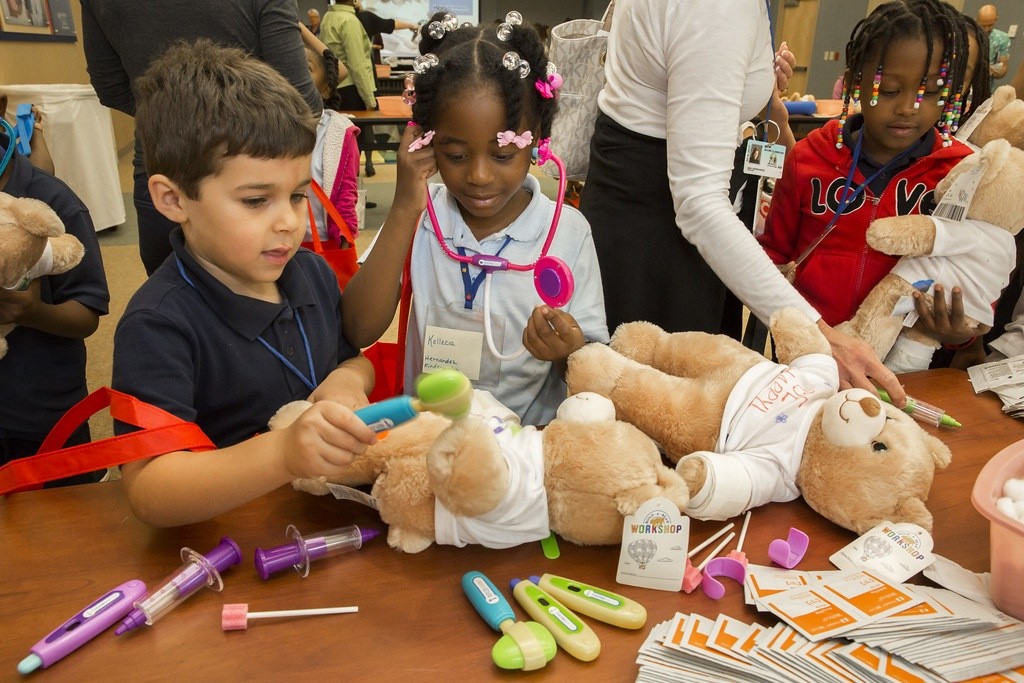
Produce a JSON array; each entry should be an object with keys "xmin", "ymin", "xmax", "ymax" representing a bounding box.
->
[
  {"xmin": 0, "ymin": 367, "xmax": 1024, "ymax": 683},
  {"xmin": 337, "ymin": 64, "xmax": 414, "ymax": 151},
  {"xmin": 0, "ymin": 84, "xmax": 126, "ymax": 232},
  {"xmin": 788, "ymin": 99, "xmax": 861, "ymax": 123}
]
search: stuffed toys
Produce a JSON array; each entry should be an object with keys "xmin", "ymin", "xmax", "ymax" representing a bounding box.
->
[
  {"xmin": 566, "ymin": 319, "xmax": 953, "ymax": 538},
  {"xmin": 966, "ymin": 85, "xmax": 1024, "ymax": 151},
  {"xmin": 266, "ymin": 380, "xmax": 690, "ymax": 554},
  {"xmin": 0, "ymin": 191, "xmax": 85, "ymax": 358},
  {"xmin": 831, "ymin": 134, "xmax": 1024, "ymax": 373}
]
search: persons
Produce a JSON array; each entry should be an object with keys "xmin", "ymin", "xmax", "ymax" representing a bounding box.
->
[
  {"xmin": 305, "ymin": 8, "xmax": 321, "ymax": 34},
  {"xmin": 298, "ymin": 42, "xmax": 362, "ymax": 251},
  {"xmin": 80, "ymin": 0, "xmax": 327, "ymax": 277},
  {"xmin": 974, "ymin": 4, "xmax": 1011, "ymax": 91},
  {"xmin": 578, "ymin": 0, "xmax": 909, "ymax": 409},
  {"xmin": 755, "ymin": 0, "xmax": 998, "ymax": 366},
  {"xmin": 750, "ymin": 146, "xmax": 760, "ymax": 163},
  {"xmin": 1, "ymin": 92, "xmax": 110, "ymax": 488},
  {"xmin": 336, "ymin": 10, "xmax": 610, "ymax": 428},
  {"xmin": 318, "ymin": 0, "xmax": 419, "ymax": 177},
  {"xmin": 108, "ymin": 31, "xmax": 380, "ymax": 528}
]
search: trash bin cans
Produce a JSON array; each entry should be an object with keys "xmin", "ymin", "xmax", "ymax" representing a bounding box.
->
[{"xmin": 0, "ymin": 84, "xmax": 112, "ymax": 177}]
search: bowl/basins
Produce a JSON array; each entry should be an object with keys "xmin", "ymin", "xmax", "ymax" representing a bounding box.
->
[{"xmin": 970, "ymin": 440, "xmax": 1024, "ymax": 622}]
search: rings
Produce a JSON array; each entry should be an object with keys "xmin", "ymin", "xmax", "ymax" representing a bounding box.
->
[{"xmin": 780, "ymin": 87, "xmax": 788, "ymax": 93}]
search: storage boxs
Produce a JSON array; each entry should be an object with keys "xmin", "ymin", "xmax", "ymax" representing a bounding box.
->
[{"xmin": 969, "ymin": 438, "xmax": 1023, "ymax": 621}]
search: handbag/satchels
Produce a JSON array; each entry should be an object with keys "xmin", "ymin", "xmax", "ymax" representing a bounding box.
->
[
  {"xmin": 297, "ymin": 177, "xmax": 360, "ymax": 293},
  {"xmin": 544, "ymin": 0, "xmax": 614, "ymax": 180},
  {"xmin": 361, "ymin": 209, "xmax": 420, "ymax": 404}
]
